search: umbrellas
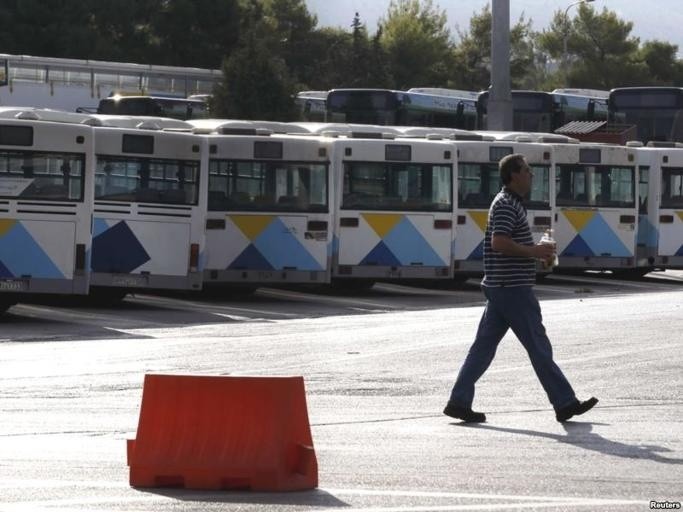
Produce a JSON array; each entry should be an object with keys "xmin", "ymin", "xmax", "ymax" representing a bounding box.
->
[
  {"xmin": 443, "ymin": 401, "xmax": 486, "ymax": 423},
  {"xmin": 554, "ymin": 396, "xmax": 599, "ymax": 422}
]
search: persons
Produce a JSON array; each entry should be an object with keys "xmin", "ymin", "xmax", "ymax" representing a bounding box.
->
[{"xmin": 442, "ymin": 153, "xmax": 598, "ymax": 424}]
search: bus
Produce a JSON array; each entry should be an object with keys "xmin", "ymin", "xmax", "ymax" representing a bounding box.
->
[{"xmin": 0, "ymin": 50, "xmax": 681, "ymax": 314}]
